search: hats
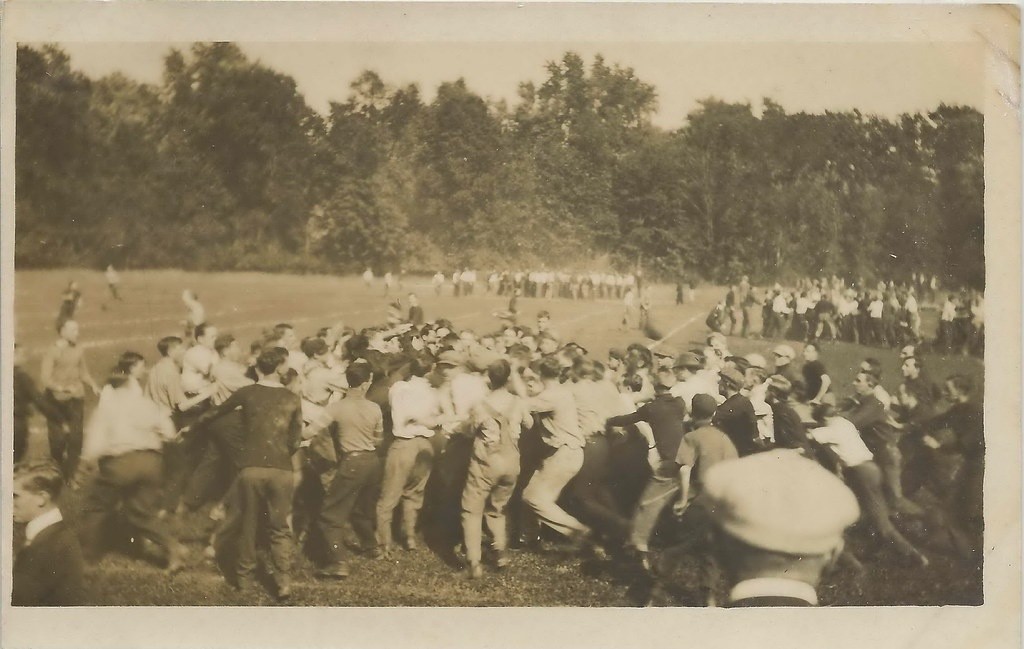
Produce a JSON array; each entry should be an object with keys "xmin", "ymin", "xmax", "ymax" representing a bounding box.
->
[
  {"xmin": 654, "ymin": 346, "xmax": 678, "ymax": 358},
  {"xmin": 773, "ymin": 346, "xmax": 795, "ymax": 359},
  {"xmin": 700, "ymin": 447, "xmax": 860, "ymax": 555},
  {"xmin": 719, "ymin": 367, "xmax": 746, "ymax": 389},
  {"xmin": 435, "ymin": 351, "xmax": 464, "ymax": 366},
  {"xmin": 676, "ymin": 352, "xmax": 700, "ymax": 367}
]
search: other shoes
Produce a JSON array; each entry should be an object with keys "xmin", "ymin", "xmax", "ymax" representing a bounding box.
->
[
  {"xmin": 494, "ymin": 551, "xmax": 507, "ymax": 567},
  {"xmin": 278, "ymin": 579, "xmax": 290, "ymax": 598},
  {"xmin": 165, "ymin": 544, "xmax": 188, "ymax": 576},
  {"xmin": 359, "ymin": 543, "xmax": 391, "ymax": 557},
  {"xmin": 316, "ymin": 560, "xmax": 349, "ymax": 579},
  {"xmin": 405, "ymin": 537, "xmax": 416, "ymax": 551},
  {"xmin": 456, "ymin": 564, "xmax": 482, "ymax": 579}
]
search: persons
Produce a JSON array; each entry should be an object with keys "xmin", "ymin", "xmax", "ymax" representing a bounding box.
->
[
  {"xmin": 100, "ymin": 261, "xmax": 121, "ymax": 308},
  {"xmin": 397, "ymin": 268, "xmax": 407, "ymax": 289},
  {"xmin": 364, "ymin": 265, "xmax": 374, "ymax": 295},
  {"xmin": 624, "ymin": 284, "xmax": 655, "ymax": 331},
  {"xmin": 433, "ymin": 270, "xmax": 445, "ymax": 295},
  {"xmin": 675, "ymin": 281, "xmax": 683, "ymax": 303},
  {"xmin": 42, "ymin": 318, "xmax": 103, "ymax": 476},
  {"xmin": 452, "ymin": 269, "xmax": 461, "ymax": 295},
  {"xmin": 382, "ymin": 270, "xmax": 393, "ymax": 297},
  {"xmin": 509, "ymin": 288, "xmax": 521, "ymax": 314},
  {"xmin": 707, "ymin": 270, "xmax": 984, "ymax": 356},
  {"xmin": 12, "ymin": 291, "xmax": 985, "ymax": 606},
  {"xmin": 57, "ymin": 281, "xmax": 82, "ymax": 329},
  {"xmin": 461, "ymin": 266, "xmax": 477, "ymax": 295},
  {"xmin": 180, "ymin": 288, "xmax": 204, "ymax": 336},
  {"xmin": 485, "ymin": 264, "xmax": 644, "ymax": 298},
  {"xmin": 688, "ymin": 278, "xmax": 696, "ymax": 301}
]
